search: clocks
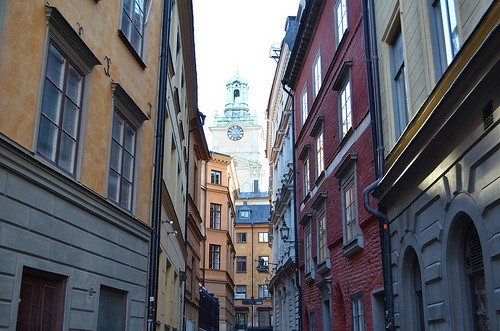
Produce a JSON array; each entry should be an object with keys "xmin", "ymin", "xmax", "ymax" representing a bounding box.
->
[{"xmin": 227, "ymin": 125, "xmax": 244, "ymax": 141}]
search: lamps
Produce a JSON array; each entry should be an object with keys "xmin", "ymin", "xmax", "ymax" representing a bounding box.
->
[
  {"xmin": 162, "ymin": 219, "xmax": 173, "ymax": 224},
  {"xmin": 168, "ymin": 231, "xmax": 178, "ymax": 236},
  {"xmin": 278, "ymin": 221, "xmax": 296, "ymax": 243}
]
